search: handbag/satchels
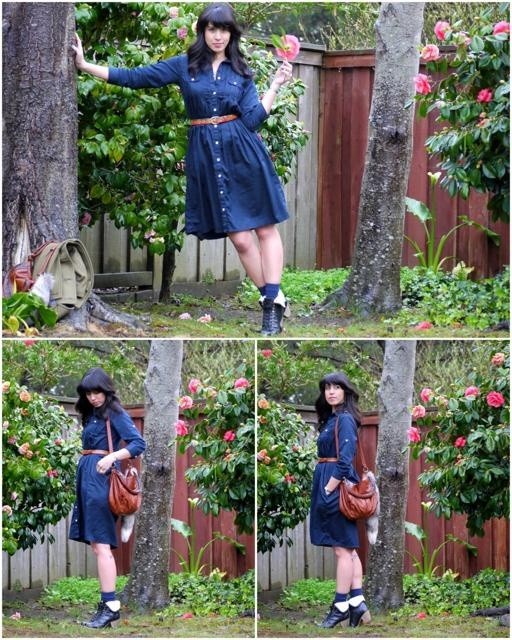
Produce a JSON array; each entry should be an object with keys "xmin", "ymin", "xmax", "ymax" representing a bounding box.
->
[
  {"xmin": 339, "ymin": 471, "xmax": 381, "ymax": 520},
  {"xmin": 108, "ymin": 468, "xmax": 143, "ymax": 516},
  {"xmin": 2, "ymin": 260, "xmax": 33, "ymax": 302}
]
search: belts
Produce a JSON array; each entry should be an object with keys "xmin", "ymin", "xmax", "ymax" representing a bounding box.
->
[
  {"xmin": 316, "ymin": 458, "xmax": 337, "ymax": 463},
  {"xmin": 79, "ymin": 449, "xmax": 109, "ymax": 455},
  {"xmin": 190, "ymin": 114, "xmax": 237, "ymax": 126}
]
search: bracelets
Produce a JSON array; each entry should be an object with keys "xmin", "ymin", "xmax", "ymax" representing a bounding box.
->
[
  {"xmin": 269, "ymin": 85, "xmax": 279, "ymax": 94},
  {"xmin": 111, "ymin": 453, "xmax": 119, "ymax": 461},
  {"xmin": 325, "ymin": 487, "xmax": 332, "ymax": 493}
]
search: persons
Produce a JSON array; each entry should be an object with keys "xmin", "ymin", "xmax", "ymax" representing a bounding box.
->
[
  {"xmin": 310, "ymin": 370, "xmax": 378, "ymax": 629},
  {"xmin": 71, "ymin": 2, "xmax": 291, "ymax": 337},
  {"xmin": 68, "ymin": 365, "xmax": 147, "ymax": 628}
]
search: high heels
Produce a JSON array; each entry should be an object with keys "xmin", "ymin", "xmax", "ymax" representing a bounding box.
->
[
  {"xmin": 80, "ymin": 600, "xmax": 121, "ymax": 630},
  {"xmin": 259, "ymin": 295, "xmax": 291, "ymax": 337},
  {"xmin": 320, "ymin": 595, "xmax": 372, "ymax": 629}
]
